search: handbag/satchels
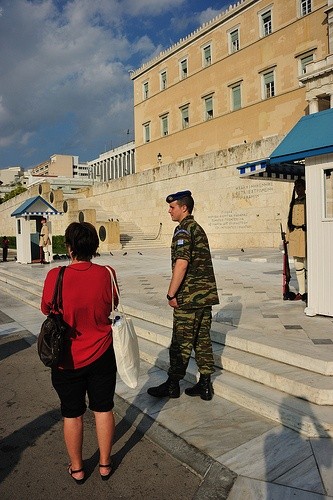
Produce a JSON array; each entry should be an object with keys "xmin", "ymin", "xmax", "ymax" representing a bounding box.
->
[
  {"xmin": 104, "ymin": 265, "xmax": 140, "ymax": 389},
  {"xmin": 43, "ymin": 245, "xmax": 53, "ymax": 262},
  {"xmin": 38, "ymin": 265, "xmax": 67, "ymax": 368}
]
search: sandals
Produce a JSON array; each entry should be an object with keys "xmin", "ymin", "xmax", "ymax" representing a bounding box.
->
[
  {"xmin": 67, "ymin": 462, "xmax": 85, "ymax": 485},
  {"xmin": 99, "ymin": 464, "xmax": 112, "ymax": 480}
]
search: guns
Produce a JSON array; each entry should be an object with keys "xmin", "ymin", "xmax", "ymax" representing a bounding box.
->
[
  {"xmin": 39, "ymin": 231, "xmax": 44, "ymax": 263},
  {"xmin": 279, "ymin": 223, "xmax": 291, "ymax": 300}
]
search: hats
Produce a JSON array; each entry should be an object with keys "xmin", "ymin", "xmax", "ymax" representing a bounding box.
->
[{"xmin": 166, "ymin": 191, "xmax": 191, "ymax": 203}]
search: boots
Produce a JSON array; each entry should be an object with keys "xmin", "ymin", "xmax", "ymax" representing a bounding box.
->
[
  {"xmin": 185, "ymin": 374, "xmax": 212, "ymax": 400},
  {"xmin": 148, "ymin": 376, "xmax": 180, "ymax": 398}
]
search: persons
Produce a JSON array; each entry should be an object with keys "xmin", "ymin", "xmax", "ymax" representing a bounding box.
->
[
  {"xmin": 285, "ymin": 179, "xmax": 306, "ymax": 301},
  {"xmin": 41, "ymin": 222, "xmax": 120, "ymax": 485},
  {"xmin": 2, "ymin": 236, "xmax": 9, "ymax": 262},
  {"xmin": 39, "ymin": 218, "xmax": 51, "ymax": 262},
  {"xmin": 147, "ymin": 190, "xmax": 220, "ymax": 400}
]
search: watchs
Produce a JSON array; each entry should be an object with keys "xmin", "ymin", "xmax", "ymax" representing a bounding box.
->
[{"xmin": 167, "ymin": 294, "xmax": 175, "ymax": 300}]
